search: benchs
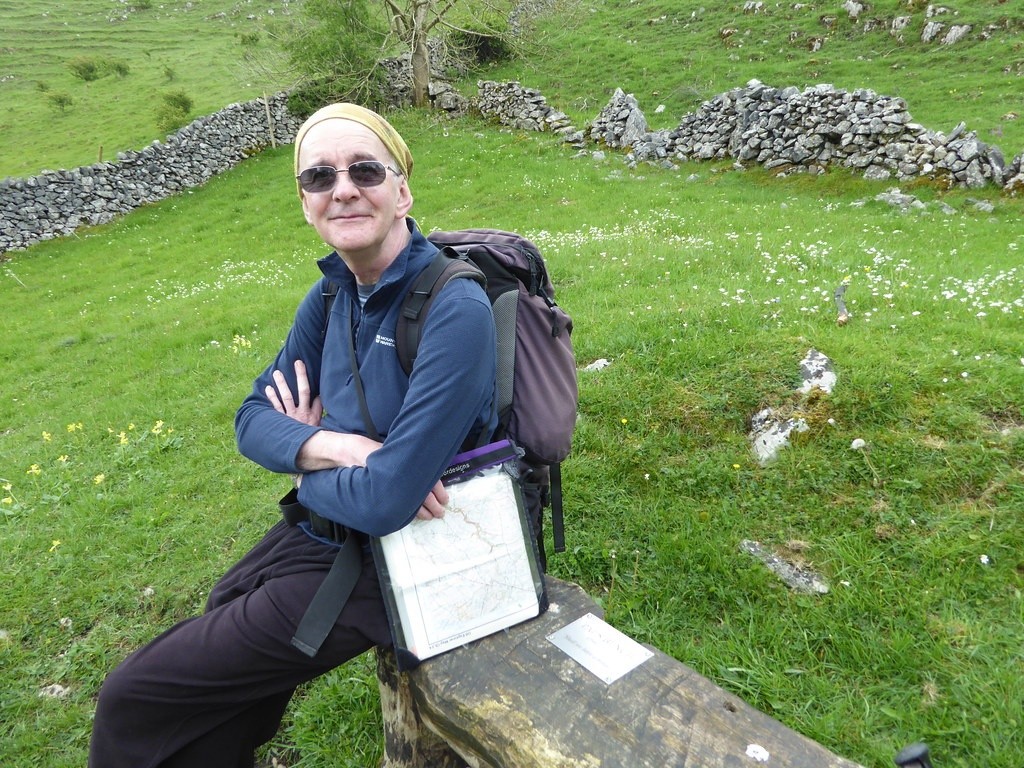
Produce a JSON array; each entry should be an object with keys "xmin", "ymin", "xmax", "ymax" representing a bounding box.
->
[{"xmin": 374, "ymin": 573, "xmax": 870, "ymax": 768}]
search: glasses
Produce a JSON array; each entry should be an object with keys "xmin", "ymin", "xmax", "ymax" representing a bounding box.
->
[{"xmin": 295, "ymin": 161, "xmax": 399, "ymax": 193}]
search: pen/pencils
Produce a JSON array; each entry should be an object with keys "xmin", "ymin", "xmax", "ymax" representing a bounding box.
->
[{"xmin": 442, "ymin": 473, "xmax": 477, "ymax": 486}]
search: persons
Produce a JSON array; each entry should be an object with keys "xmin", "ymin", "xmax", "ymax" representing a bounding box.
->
[{"xmin": 88, "ymin": 102, "xmax": 547, "ymax": 768}]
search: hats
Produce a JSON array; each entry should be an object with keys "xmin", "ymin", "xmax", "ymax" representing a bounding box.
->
[{"xmin": 295, "ymin": 103, "xmax": 414, "ymax": 202}]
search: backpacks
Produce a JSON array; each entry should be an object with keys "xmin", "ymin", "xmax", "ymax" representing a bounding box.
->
[{"xmin": 321, "ymin": 229, "xmax": 579, "ymax": 573}]
